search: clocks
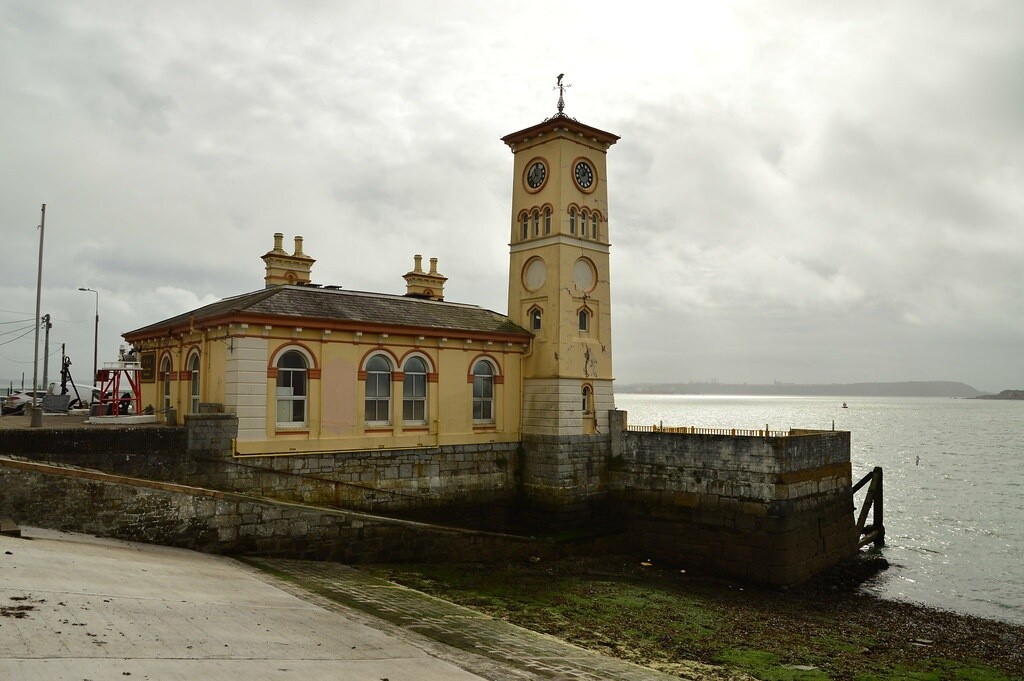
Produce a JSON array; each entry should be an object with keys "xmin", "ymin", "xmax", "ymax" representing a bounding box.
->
[
  {"xmin": 571, "ymin": 156, "xmax": 599, "ymax": 195},
  {"xmin": 522, "ymin": 156, "xmax": 550, "ymax": 195}
]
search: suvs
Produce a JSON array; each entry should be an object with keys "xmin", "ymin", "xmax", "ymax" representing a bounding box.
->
[{"xmin": 4, "ymin": 390, "xmax": 47, "ymax": 415}]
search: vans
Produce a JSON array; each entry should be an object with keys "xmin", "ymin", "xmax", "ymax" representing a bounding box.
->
[{"xmin": 45, "ymin": 381, "xmax": 133, "ymax": 415}]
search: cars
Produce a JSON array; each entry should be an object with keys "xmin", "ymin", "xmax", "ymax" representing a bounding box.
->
[{"xmin": 0, "ymin": 395, "xmax": 8, "ymax": 408}]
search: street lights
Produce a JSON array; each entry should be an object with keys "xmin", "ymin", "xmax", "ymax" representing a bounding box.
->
[{"xmin": 79, "ymin": 289, "xmax": 99, "ymax": 388}]
